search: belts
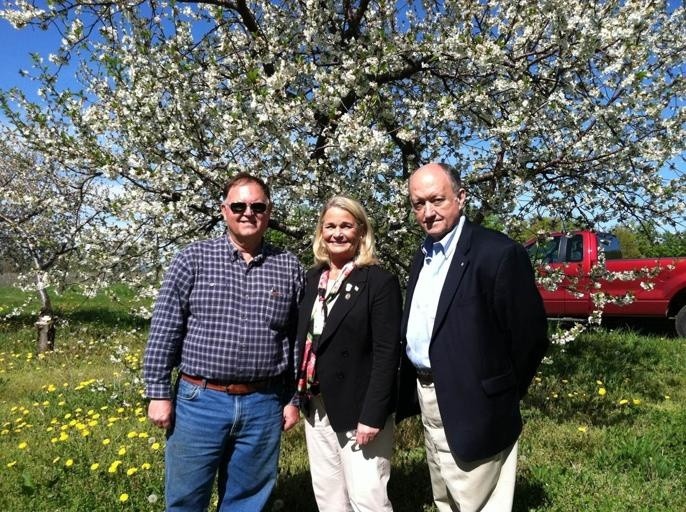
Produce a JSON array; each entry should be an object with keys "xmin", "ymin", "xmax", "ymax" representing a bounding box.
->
[
  {"xmin": 180, "ymin": 372, "xmax": 268, "ymax": 396},
  {"xmin": 414, "ymin": 369, "xmax": 434, "ymax": 386}
]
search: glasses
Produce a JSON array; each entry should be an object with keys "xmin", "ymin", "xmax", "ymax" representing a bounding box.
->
[{"xmin": 225, "ymin": 201, "xmax": 269, "ymax": 213}]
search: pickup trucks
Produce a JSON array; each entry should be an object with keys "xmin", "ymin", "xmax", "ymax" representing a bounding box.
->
[{"xmin": 523, "ymin": 230, "xmax": 686, "ymax": 339}]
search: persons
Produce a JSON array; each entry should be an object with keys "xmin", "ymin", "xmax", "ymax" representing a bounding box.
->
[
  {"xmin": 395, "ymin": 161, "xmax": 550, "ymax": 512},
  {"xmin": 142, "ymin": 174, "xmax": 302, "ymax": 512},
  {"xmin": 293, "ymin": 196, "xmax": 404, "ymax": 512}
]
